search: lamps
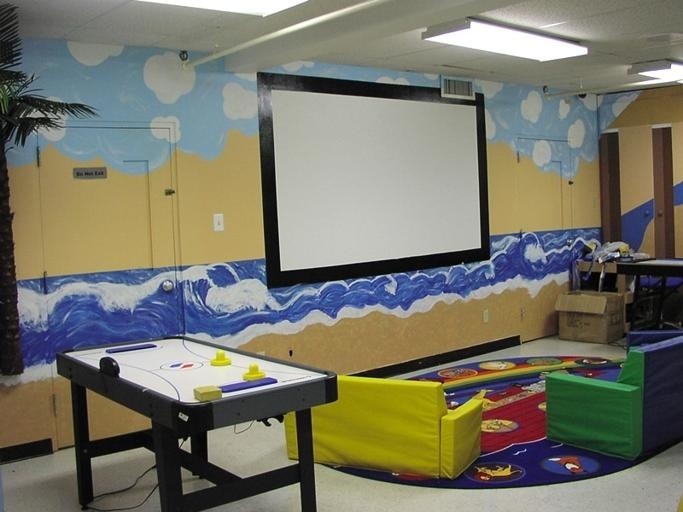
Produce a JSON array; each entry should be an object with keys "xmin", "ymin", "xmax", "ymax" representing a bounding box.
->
[
  {"xmin": 420, "ymin": 18, "xmax": 589, "ymax": 64},
  {"xmin": 626, "ymin": 58, "xmax": 682, "ymax": 84}
]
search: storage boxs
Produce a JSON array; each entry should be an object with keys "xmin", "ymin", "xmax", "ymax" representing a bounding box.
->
[{"xmin": 552, "ymin": 289, "xmax": 625, "ymax": 345}]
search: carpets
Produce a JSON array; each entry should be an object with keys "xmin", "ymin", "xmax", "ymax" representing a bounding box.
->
[{"xmin": 313, "ymin": 354, "xmax": 682, "ymax": 490}]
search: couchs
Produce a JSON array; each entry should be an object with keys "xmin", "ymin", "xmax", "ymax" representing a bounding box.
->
[
  {"xmin": 283, "ymin": 373, "xmax": 488, "ymax": 482},
  {"xmin": 544, "ymin": 330, "xmax": 682, "ymax": 462}
]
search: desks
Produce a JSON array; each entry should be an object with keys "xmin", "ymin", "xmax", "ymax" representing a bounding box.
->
[
  {"xmin": 617, "ymin": 256, "xmax": 683, "ymax": 331},
  {"xmin": 54, "ymin": 335, "xmax": 339, "ymax": 512}
]
